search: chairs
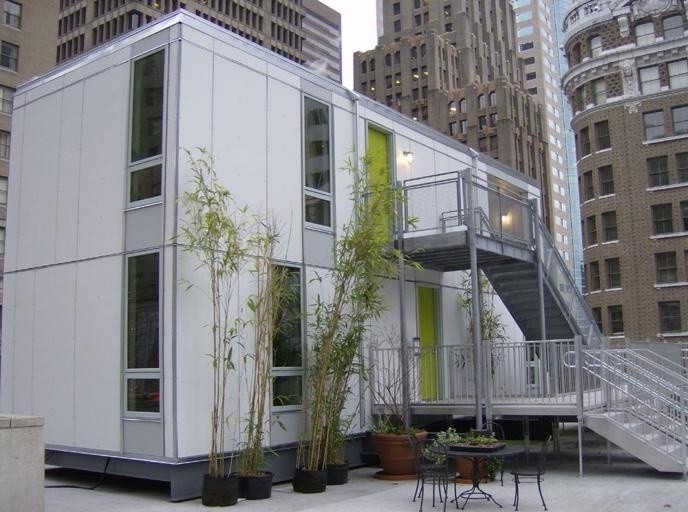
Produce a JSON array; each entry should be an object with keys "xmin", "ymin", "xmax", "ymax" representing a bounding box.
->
[
  {"xmin": 467, "ymin": 420, "xmax": 507, "ymax": 487},
  {"xmin": 413, "ymin": 438, "xmax": 460, "ymax": 512},
  {"xmin": 406, "ymin": 431, "xmax": 450, "ymax": 511},
  {"xmin": 510, "ymin": 434, "xmax": 554, "ymax": 512}
]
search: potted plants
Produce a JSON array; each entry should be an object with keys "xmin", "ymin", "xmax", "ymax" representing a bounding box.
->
[
  {"xmin": 147, "ymin": 144, "xmax": 241, "ymax": 507},
  {"xmin": 238, "ymin": 206, "xmax": 313, "ymax": 502},
  {"xmin": 292, "ymin": 146, "xmax": 426, "ymax": 495}
]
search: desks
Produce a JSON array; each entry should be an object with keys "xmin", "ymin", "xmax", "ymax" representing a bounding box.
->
[{"xmin": 427, "ymin": 435, "xmax": 529, "ymax": 510}]
